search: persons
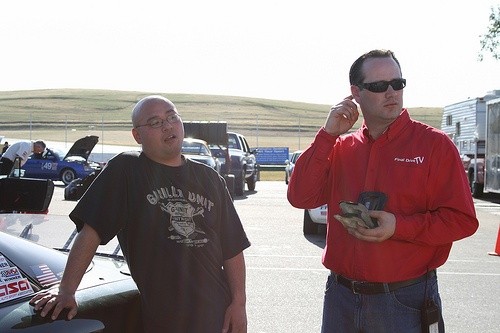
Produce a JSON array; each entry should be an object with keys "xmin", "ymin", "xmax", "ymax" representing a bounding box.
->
[
  {"xmin": 2, "ymin": 142, "xmax": 8, "ymax": 154},
  {"xmin": 30, "ymin": 96, "xmax": 251, "ymax": 332},
  {"xmin": 0, "ymin": 140, "xmax": 46, "ymax": 175},
  {"xmin": 288, "ymin": 49, "xmax": 478, "ymax": 332}
]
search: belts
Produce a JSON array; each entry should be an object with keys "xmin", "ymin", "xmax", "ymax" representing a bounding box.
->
[{"xmin": 330, "ymin": 268, "xmax": 439, "ymax": 294}]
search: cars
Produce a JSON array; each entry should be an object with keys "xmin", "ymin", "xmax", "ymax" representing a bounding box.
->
[
  {"xmin": 177, "ymin": 119, "xmax": 234, "ymax": 202},
  {"xmin": 284, "ymin": 151, "xmax": 304, "ymax": 184},
  {"xmin": 20, "ymin": 135, "xmax": 101, "ymax": 185},
  {"xmin": 303, "ymin": 204, "xmax": 328, "ymax": 235},
  {"xmin": 209, "ymin": 132, "xmax": 256, "ymax": 199}
]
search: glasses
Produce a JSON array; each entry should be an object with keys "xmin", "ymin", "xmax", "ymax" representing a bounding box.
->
[
  {"xmin": 135, "ymin": 111, "xmax": 181, "ymax": 128},
  {"xmin": 358, "ymin": 78, "xmax": 407, "ymax": 92}
]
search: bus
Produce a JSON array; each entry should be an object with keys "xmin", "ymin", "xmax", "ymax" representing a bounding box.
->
[{"xmin": 0, "ymin": 209, "xmax": 144, "ymax": 333}]
